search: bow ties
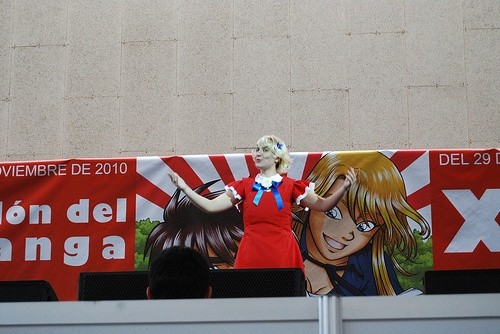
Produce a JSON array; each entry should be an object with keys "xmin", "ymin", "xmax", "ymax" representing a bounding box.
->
[{"xmin": 253, "ymin": 180, "xmax": 284, "ymax": 210}]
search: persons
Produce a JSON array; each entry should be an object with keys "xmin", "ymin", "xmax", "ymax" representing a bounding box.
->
[{"xmin": 166, "ymin": 135, "xmax": 361, "ymax": 269}]
